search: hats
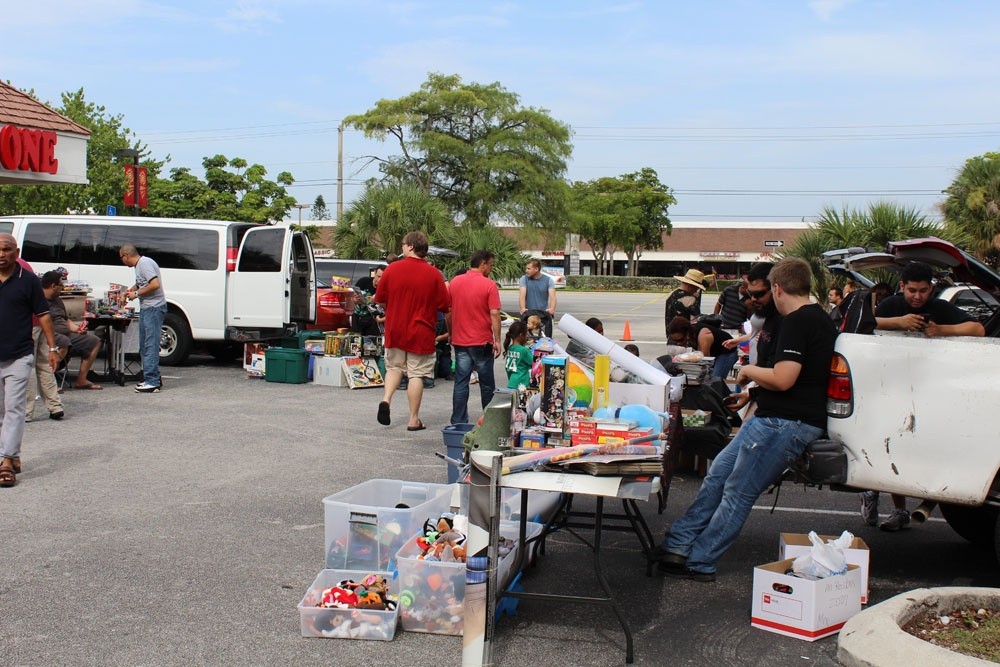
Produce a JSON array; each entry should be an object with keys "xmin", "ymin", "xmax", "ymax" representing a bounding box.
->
[{"xmin": 673, "ymin": 268, "xmax": 708, "ymax": 292}]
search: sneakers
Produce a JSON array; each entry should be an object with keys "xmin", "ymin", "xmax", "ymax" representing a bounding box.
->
[{"xmin": 135, "ymin": 378, "xmax": 163, "ymax": 392}]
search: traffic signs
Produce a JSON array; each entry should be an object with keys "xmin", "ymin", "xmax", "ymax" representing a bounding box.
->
[{"xmin": 765, "ymin": 239, "xmax": 785, "ymax": 247}]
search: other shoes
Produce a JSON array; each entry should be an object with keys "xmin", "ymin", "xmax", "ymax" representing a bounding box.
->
[
  {"xmin": 656, "ymin": 564, "xmax": 716, "ymax": 582},
  {"xmin": 641, "ymin": 544, "xmax": 682, "ymax": 568},
  {"xmin": 49, "ymin": 411, "xmax": 64, "ymax": 418}
]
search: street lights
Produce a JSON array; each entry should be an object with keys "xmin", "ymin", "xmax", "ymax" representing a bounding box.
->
[
  {"xmin": 111, "ymin": 147, "xmax": 140, "ymax": 217},
  {"xmin": 292, "ymin": 204, "xmax": 310, "ymax": 229}
]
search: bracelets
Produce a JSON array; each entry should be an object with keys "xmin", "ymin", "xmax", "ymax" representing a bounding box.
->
[
  {"xmin": 49, "ymin": 346, "xmax": 58, "ymax": 353},
  {"xmin": 134, "ymin": 289, "xmax": 139, "ymax": 299}
]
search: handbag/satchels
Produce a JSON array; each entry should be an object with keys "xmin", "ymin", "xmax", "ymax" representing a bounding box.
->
[
  {"xmin": 831, "ymin": 288, "xmax": 878, "ymax": 333},
  {"xmin": 692, "ymin": 314, "xmax": 723, "ymax": 328}
]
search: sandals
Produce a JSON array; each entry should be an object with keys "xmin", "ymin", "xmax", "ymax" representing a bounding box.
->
[{"xmin": 0, "ymin": 459, "xmax": 21, "ymax": 486}]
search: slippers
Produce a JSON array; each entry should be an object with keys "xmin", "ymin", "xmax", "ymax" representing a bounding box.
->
[
  {"xmin": 377, "ymin": 401, "xmax": 391, "ymax": 425},
  {"xmin": 74, "ymin": 382, "xmax": 103, "ymax": 389},
  {"xmin": 407, "ymin": 418, "xmax": 426, "ymax": 431}
]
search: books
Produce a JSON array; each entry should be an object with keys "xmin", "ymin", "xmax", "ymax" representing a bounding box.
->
[{"xmin": 546, "ymin": 419, "xmax": 666, "ymax": 476}]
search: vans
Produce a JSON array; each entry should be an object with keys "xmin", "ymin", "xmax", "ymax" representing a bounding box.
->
[
  {"xmin": 312, "ymin": 245, "xmax": 461, "ymax": 299},
  {"xmin": 1, "ymin": 213, "xmax": 318, "ymax": 367}
]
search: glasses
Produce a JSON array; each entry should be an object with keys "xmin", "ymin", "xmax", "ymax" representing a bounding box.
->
[
  {"xmin": 745, "ymin": 286, "xmax": 770, "ymax": 298},
  {"xmin": 119, "ymin": 254, "xmax": 128, "ymax": 260},
  {"xmin": 400, "ymin": 241, "xmax": 407, "ymax": 246}
]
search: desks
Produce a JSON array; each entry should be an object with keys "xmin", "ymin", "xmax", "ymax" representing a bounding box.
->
[
  {"xmin": 85, "ymin": 308, "xmax": 140, "ymax": 387},
  {"xmin": 677, "ymin": 377, "xmax": 733, "ymax": 478}
]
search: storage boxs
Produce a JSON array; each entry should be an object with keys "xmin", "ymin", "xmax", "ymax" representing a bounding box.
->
[
  {"xmin": 517, "ymin": 406, "xmax": 657, "ymax": 456},
  {"xmin": 326, "ymin": 328, "xmax": 383, "ymax": 359},
  {"xmin": 341, "ymin": 356, "xmax": 386, "ymax": 390},
  {"xmin": 244, "ymin": 344, "xmax": 270, "ymax": 372},
  {"xmin": 264, "ymin": 349, "xmax": 311, "ymax": 385},
  {"xmin": 683, "ymin": 408, "xmax": 713, "ymax": 427},
  {"xmin": 751, "ymin": 558, "xmax": 863, "ymax": 645},
  {"xmin": 589, "ymin": 376, "xmax": 672, "ymax": 434},
  {"xmin": 531, "ymin": 338, "xmax": 554, "ymax": 388},
  {"xmin": 540, "ymin": 355, "xmax": 572, "ymax": 433},
  {"xmin": 313, "ymin": 356, "xmax": 349, "ymax": 388},
  {"xmin": 779, "ymin": 532, "xmax": 870, "ymax": 605},
  {"xmin": 375, "ymin": 356, "xmax": 387, "ymax": 383},
  {"xmin": 566, "ymin": 340, "xmax": 632, "ymax": 384},
  {"xmin": 305, "ymin": 340, "xmax": 326, "ymax": 353},
  {"xmin": 323, "ymin": 477, "xmax": 457, "ymax": 573},
  {"xmin": 92, "ymin": 283, "xmax": 136, "ymax": 317},
  {"xmin": 297, "ymin": 568, "xmax": 402, "ymax": 643},
  {"xmin": 394, "ymin": 517, "xmax": 544, "ymax": 639},
  {"xmin": 60, "ymin": 295, "xmax": 94, "ymax": 320}
]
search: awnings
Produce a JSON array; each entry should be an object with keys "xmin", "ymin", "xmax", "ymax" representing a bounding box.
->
[{"xmin": 501, "ymin": 241, "xmax": 786, "ymax": 272}]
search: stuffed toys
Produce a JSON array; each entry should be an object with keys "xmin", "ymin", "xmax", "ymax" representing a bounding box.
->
[{"xmin": 305, "ymin": 507, "xmax": 519, "ymax": 640}]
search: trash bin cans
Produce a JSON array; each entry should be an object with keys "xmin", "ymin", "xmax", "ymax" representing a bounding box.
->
[{"xmin": 440, "ymin": 422, "xmax": 477, "ymax": 483}]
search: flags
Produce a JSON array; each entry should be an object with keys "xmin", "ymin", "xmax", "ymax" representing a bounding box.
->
[
  {"xmin": 137, "ymin": 166, "xmax": 148, "ymax": 208},
  {"xmin": 125, "ymin": 163, "xmax": 135, "ymax": 205}
]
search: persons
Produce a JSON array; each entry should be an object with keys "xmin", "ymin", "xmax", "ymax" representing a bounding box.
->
[
  {"xmin": 852, "ymin": 258, "xmax": 987, "ymax": 531},
  {"xmin": 445, "ymin": 249, "xmax": 503, "ymax": 428},
  {"xmin": 525, "ymin": 316, "xmax": 547, "ymax": 345},
  {"xmin": 0, "ymin": 232, "xmax": 60, "ymax": 488},
  {"xmin": 15, "ymin": 248, "xmax": 66, "ymax": 423},
  {"xmin": 608, "ymin": 342, "xmax": 644, "ymax": 383},
  {"xmin": 520, "ymin": 258, "xmax": 558, "ymax": 316},
  {"xmin": 118, "ymin": 242, "xmax": 169, "ymax": 394},
  {"xmin": 564, "ymin": 316, "xmax": 605, "ymax": 371},
  {"xmin": 40, "ymin": 271, "xmax": 105, "ymax": 393},
  {"xmin": 643, "ymin": 257, "xmax": 842, "ymax": 587},
  {"xmin": 656, "ymin": 267, "xmax": 896, "ymax": 415},
  {"xmin": 503, "ymin": 320, "xmax": 537, "ymax": 391},
  {"xmin": 349, "ymin": 253, "xmax": 468, "ymax": 391},
  {"xmin": 376, "ymin": 232, "xmax": 452, "ymax": 432}
]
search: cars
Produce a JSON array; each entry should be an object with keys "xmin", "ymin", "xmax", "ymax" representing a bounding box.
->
[
  {"xmin": 745, "ymin": 231, "xmax": 1000, "ymax": 546},
  {"xmin": 307, "ymin": 276, "xmax": 365, "ymax": 334}
]
